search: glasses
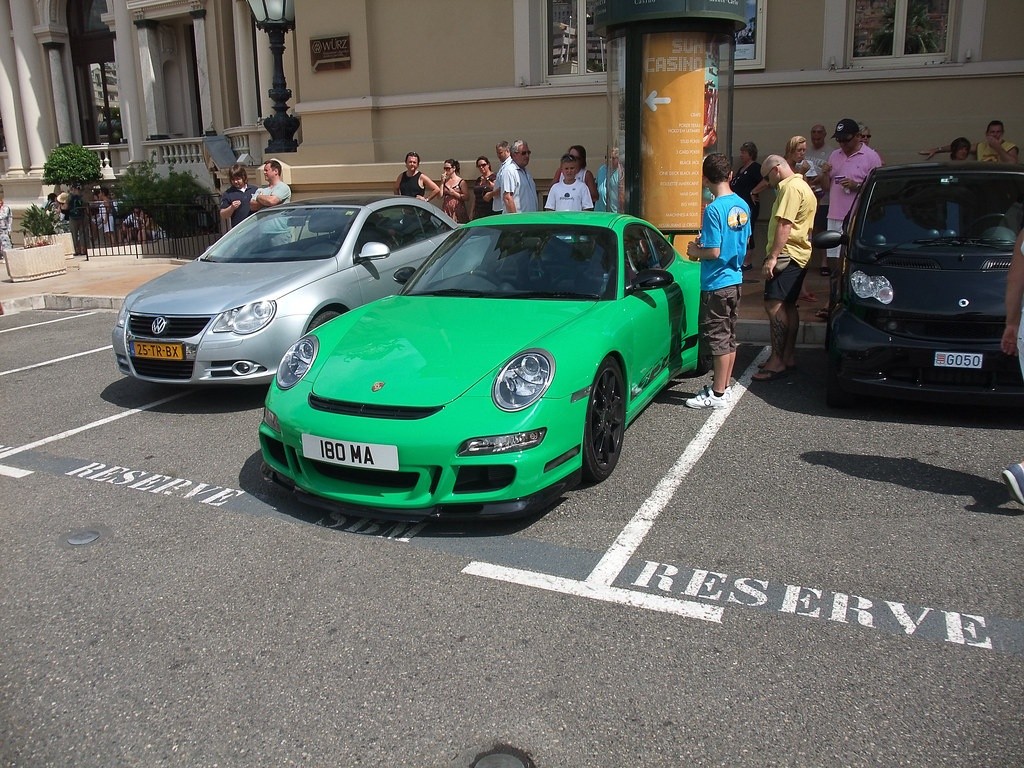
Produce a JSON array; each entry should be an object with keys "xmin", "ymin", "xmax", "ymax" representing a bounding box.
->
[
  {"xmin": 444, "ymin": 166, "xmax": 454, "ymax": 171},
  {"xmin": 860, "ymin": 134, "xmax": 872, "ymax": 138},
  {"xmin": 763, "ymin": 164, "xmax": 780, "ymax": 182},
  {"xmin": 835, "ymin": 135, "xmax": 855, "ymax": 144},
  {"xmin": 478, "ymin": 163, "xmax": 488, "ymax": 168},
  {"xmin": 605, "ymin": 155, "xmax": 617, "ymax": 160},
  {"xmin": 514, "ymin": 151, "xmax": 531, "ymax": 156}
]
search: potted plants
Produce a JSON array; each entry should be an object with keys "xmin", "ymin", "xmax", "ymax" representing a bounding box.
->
[{"xmin": 20, "ymin": 203, "xmax": 75, "ymax": 260}]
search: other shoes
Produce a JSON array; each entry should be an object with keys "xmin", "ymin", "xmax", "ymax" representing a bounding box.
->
[
  {"xmin": 741, "ymin": 263, "xmax": 752, "ymax": 271},
  {"xmin": 1002, "ymin": 464, "xmax": 1024, "ymax": 505},
  {"xmin": 815, "ymin": 308, "xmax": 828, "ymax": 318},
  {"xmin": 821, "ymin": 266, "xmax": 828, "ymax": 274},
  {"xmin": 73, "ymin": 252, "xmax": 86, "ymax": 256}
]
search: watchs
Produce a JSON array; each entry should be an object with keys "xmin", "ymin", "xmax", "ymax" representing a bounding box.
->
[{"xmin": 854, "ymin": 182, "xmax": 861, "ymax": 189}]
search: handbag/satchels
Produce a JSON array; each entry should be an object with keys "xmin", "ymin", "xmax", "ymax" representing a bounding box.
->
[
  {"xmin": 474, "ymin": 185, "xmax": 492, "ymax": 202},
  {"xmin": 455, "ymin": 201, "xmax": 469, "ymax": 222}
]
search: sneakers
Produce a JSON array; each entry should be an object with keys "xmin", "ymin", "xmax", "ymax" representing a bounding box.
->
[{"xmin": 686, "ymin": 385, "xmax": 736, "ymax": 409}]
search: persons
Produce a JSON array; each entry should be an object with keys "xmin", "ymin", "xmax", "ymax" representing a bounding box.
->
[
  {"xmin": 950, "ymin": 137, "xmax": 971, "ymax": 161},
  {"xmin": 0, "ymin": 200, "xmax": 13, "ymax": 263},
  {"xmin": 783, "ymin": 118, "xmax": 881, "ymax": 319},
  {"xmin": 46, "ymin": 187, "xmax": 164, "ymax": 255},
  {"xmin": 702, "ymin": 141, "xmax": 768, "ymax": 271},
  {"xmin": 750, "ymin": 154, "xmax": 817, "ymax": 381},
  {"xmin": 686, "ymin": 151, "xmax": 752, "ymax": 409},
  {"xmin": 394, "ymin": 139, "xmax": 539, "ymax": 224},
  {"xmin": 545, "ymin": 145, "xmax": 623, "ymax": 212},
  {"xmin": 250, "ymin": 161, "xmax": 292, "ymax": 247},
  {"xmin": 736, "ymin": 18, "xmax": 755, "ymax": 44},
  {"xmin": 219, "ymin": 166, "xmax": 258, "ymax": 241},
  {"xmin": 1001, "ymin": 229, "xmax": 1024, "ymax": 505},
  {"xmin": 920, "ymin": 120, "xmax": 1019, "ymax": 164}
]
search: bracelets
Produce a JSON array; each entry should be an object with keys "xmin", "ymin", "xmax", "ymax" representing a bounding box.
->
[
  {"xmin": 750, "ymin": 192, "xmax": 758, "ymax": 195},
  {"xmin": 425, "ymin": 197, "xmax": 428, "ymax": 202},
  {"xmin": 938, "ymin": 147, "xmax": 942, "ymax": 152}
]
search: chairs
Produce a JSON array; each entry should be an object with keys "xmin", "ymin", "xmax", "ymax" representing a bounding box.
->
[{"xmin": 308, "ymin": 213, "xmax": 336, "ymax": 243}]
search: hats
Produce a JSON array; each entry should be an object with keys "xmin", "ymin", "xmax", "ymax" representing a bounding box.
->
[
  {"xmin": 831, "ymin": 119, "xmax": 859, "ymax": 139},
  {"xmin": 56, "ymin": 193, "xmax": 70, "ymax": 203}
]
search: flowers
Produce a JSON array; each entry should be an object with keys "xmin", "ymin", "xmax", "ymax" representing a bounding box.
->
[{"xmin": 24, "ymin": 231, "xmax": 57, "ymax": 249}]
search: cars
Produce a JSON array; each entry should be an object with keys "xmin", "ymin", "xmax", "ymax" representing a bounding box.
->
[
  {"xmin": 112, "ymin": 195, "xmax": 493, "ymax": 385},
  {"xmin": 260, "ymin": 212, "xmax": 702, "ymax": 521},
  {"xmin": 811, "ymin": 164, "xmax": 1024, "ymax": 410}
]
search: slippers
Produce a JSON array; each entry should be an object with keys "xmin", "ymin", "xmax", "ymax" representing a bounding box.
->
[
  {"xmin": 751, "ymin": 368, "xmax": 788, "ymax": 380},
  {"xmin": 799, "ymin": 293, "xmax": 818, "ymax": 301},
  {"xmin": 757, "ymin": 359, "xmax": 797, "ymax": 370}
]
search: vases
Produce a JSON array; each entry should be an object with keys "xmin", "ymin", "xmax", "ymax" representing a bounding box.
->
[{"xmin": 3, "ymin": 243, "xmax": 66, "ymax": 283}]
